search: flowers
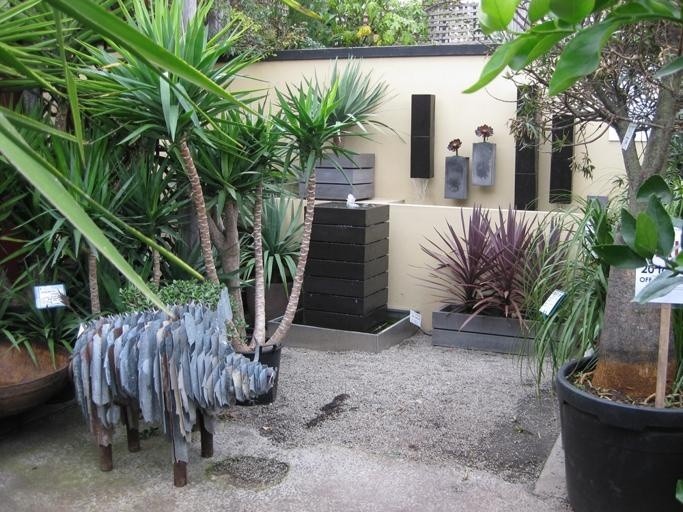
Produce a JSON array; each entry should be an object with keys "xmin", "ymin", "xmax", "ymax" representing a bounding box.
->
[
  {"xmin": 475, "ymin": 123, "xmax": 494, "ymax": 142},
  {"xmin": 446, "ymin": 139, "xmax": 461, "ymax": 155}
]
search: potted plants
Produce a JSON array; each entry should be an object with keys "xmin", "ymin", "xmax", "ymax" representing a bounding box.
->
[
  {"xmin": 0, "ymin": 0, "xmax": 380, "ymax": 407},
  {"xmin": 460, "ymin": 0, "xmax": 683, "ymax": 512},
  {"xmin": 405, "ymin": 201, "xmax": 575, "ymax": 359},
  {"xmin": 275, "ymin": 53, "xmax": 407, "ymax": 200}
]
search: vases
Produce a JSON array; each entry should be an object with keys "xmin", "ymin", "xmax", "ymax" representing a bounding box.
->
[
  {"xmin": 444, "ymin": 156, "xmax": 469, "ymax": 201},
  {"xmin": 301, "ymin": 201, "xmax": 392, "ymax": 334},
  {"xmin": 471, "ymin": 143, "xmax": 496, "ymax": 186}
]
